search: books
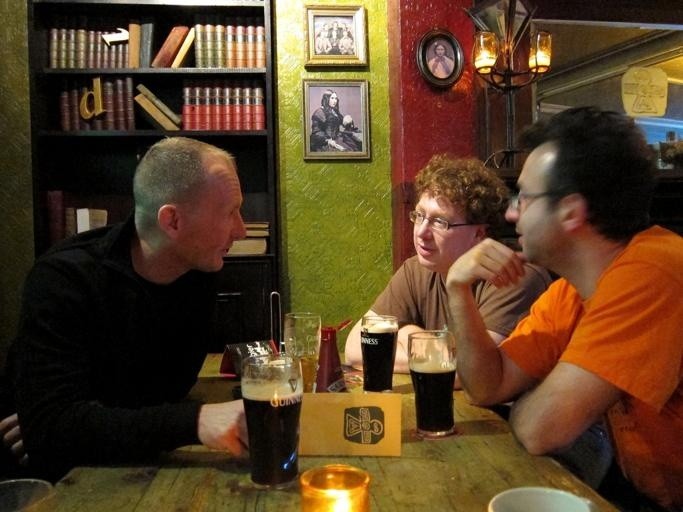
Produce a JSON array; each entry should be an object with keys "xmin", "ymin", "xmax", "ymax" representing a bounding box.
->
[
  {"xmin": 50, "ymin": 24, "xmax": 140, "ymax": 69},
  {"xmin": 229, "ymin": 221, "xmax": 270, "ymax": 255},
  {"xmin": 151, "ymin": 24, "xmax": 265, "ymax": 68},
  {"xmin": 46, "ymin": 190, "xmax": 109, "ymax": 244},
  {"xmin": 134, "ymin": 84, "xmax": 181, "ymax": 131},
  {"xmin": 182, "ymin": 87, "xmax": 265, "ymax": 131},
  {"xmin": 60, "ymin": 78, "xmax": 133, "ymax": 131}
]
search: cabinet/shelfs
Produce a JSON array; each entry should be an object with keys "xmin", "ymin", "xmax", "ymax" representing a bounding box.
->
[{"xmin": 27, "ymin": 0, "xmax": 278, "ymax": 349}]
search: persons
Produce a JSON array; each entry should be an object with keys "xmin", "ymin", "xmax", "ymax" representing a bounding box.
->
[
  {"xmin": 314, "ymin": 20, "xmax": 355, "ymax": 55},
  {"xmin": 344, "ymin": 154, "xmax": 612, "ymax": 492},
  {"xmin": 0, "ymin": 136, "xmax": 250, "ymax": 479},
  {"xmin": 429, "ymin": 44, "xmax": 455, "ymax": 79},
  {"xmin": 310, "ymin": 90, "xmax": 358, "ymax": 152},
  {"xmin": 446, "ymin": 107, "xmax": 683, "ymax": 512}
]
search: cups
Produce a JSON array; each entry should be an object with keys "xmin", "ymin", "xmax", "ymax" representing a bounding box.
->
[
  {"xmin": 407, "ymin": 330, "xmax": 457, "ymax": 436},
  {"xmin": 241, "ymin": 354, "xmax": 304, "ymax": 485},
  {"xmin": 0, "ymin": 479, "xmax": 53, "ymax": 512},
  {"xmin": 284, "ymin": 313, "xmax": 321, "ymax": 392},
  {"xmin": 300, "ymin": 463, "xmax": 371, "ymax": 512},
  {"xmin": 361, "ymin": 315, "xmax": 398, "ymax": 392}
]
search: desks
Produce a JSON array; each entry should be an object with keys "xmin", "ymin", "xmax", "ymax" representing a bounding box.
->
[{"xmin": 24, "ymin": 364, "xmax": 623, "ymax": 512}]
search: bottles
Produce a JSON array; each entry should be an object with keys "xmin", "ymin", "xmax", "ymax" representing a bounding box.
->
[{"xmin": 314, "ymin": 319, "xmax": 351, "ymax": 392}]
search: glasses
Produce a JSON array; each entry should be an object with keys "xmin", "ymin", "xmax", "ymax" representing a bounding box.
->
[
  {"xmin": 409, "ymin": 210, "xmax": 484, "ymax": 232},
  {"xmin": 511, "ymin": 192, "xmax": 546, "ymax": 212}
]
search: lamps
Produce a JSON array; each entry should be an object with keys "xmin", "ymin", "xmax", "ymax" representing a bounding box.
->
[{"xmin": 473, "ymin": 0, "xmax": 555, "ymax": 170}]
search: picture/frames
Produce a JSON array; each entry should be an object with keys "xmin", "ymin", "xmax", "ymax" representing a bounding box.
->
[
  {"xmin": 415, "ymin": 27, "xmax": 465, "ymax": 88},
  {"xmin": 302, "ymin": 78, "xmax": 371, "ymax": 161},
  {"xmin": 304, "ymin": 3, "xmax": 367, "ymax": 68}
]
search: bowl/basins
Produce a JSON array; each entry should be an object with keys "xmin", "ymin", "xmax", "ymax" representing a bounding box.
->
[{"xmin": 487, "ymin": 486, "xmax": 590, "ymax": 512}]
